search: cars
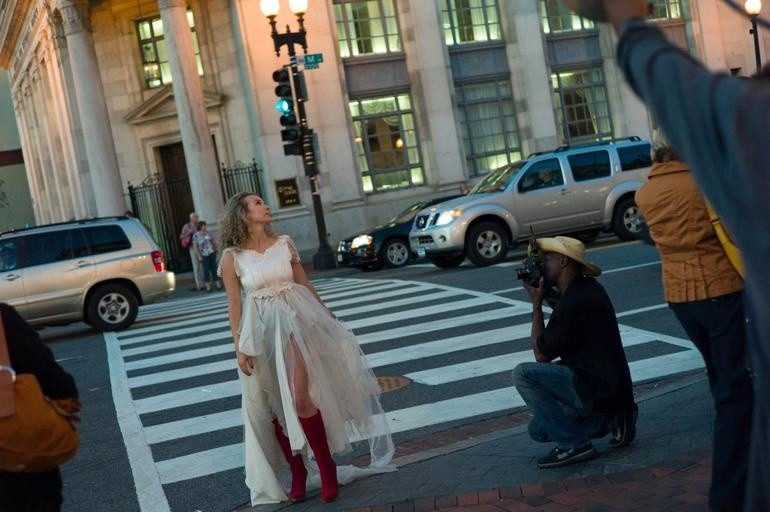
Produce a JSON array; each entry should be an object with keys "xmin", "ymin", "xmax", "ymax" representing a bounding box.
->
[{"xmin": 1, "ymin": 214, "xmax": 177, "ymax": 332}]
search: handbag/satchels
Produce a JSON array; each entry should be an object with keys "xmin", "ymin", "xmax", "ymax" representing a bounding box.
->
[
  {"xmin": 702, "ymin": 190, "xmax": 748, "ymax": 284},
  {"xmin": 0, "ymin": 312, "xmax": 80, "ymax": 475},
  {"xmin": 180, "ymin": 234, "xmax": 193, "ymax": 247}
]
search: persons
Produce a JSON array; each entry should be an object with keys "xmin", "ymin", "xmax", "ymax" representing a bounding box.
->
[
  {"xmin": 179, "ymin": 211, "xmax": 209, "ymax": 292},
  {"xmin": 633, "ymin": 121, "xmax": 751, "ymax": 511},
  {"xmin": 557, "ymin": 0, "xmax": 770, "ymax": 511},
  {"xmin": 191, "ymin": 220, "xmax": 222, "ymax": 292},
  {"xmin": 511, "ymin": 236, "xmax": 639, "ymax": 469},
  {"xmin": 124, "ymin": 211, "xmax": 134, "ymax": 217},
  {"xmin": 535, "ymin": 163, "xmax": 558, "ymax": 187},
  {"xmin": 1, "ymin": 300, "xmax": 80, "ymax": 510},
  {"xmin": 215, "ymin": 190, "xmax": 397, "ymax": 503}
]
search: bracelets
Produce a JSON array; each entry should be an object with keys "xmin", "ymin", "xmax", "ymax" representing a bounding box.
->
[{"xmin": 531, "ymin": 307, "xmax": 544, "ymax": 321}]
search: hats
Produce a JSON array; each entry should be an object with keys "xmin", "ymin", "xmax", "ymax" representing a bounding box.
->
[{"xmin": 528, "ymin": 231, "xmax": 602, "ymax": 280}]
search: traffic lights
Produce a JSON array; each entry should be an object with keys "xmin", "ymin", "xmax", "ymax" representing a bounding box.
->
[{"xmin": 273, "ymin": 70, "xmax": 303, "ymax": 158}]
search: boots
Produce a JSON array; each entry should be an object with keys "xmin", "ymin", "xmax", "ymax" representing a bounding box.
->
[
  {"xmin": 296, "ymin": 408, "xmax": 339, "ymax": 503},
  {"xmin": 265, "ymin": 413, "xmax": 310, "ymax": 502}
]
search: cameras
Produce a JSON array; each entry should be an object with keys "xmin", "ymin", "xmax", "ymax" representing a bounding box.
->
[{"xmin": 514, "ymin": 254, "xmax": 546, "ymax": 288}]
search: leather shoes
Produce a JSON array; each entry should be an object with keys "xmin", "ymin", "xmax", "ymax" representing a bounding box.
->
[
  {"xmin": 608, "ymin": 402, "xmax": 639, "ymax": 449},
  {"xmin": 215, "ymin": 284, "xmax": 222, "ymax": 291},
  {"xmin": 535, "ymin": 441, "xmax": 598, "ymax": 469},
  {"xmin": 206, "ymin": 286, "xmax": 212, "ymax": 293}
]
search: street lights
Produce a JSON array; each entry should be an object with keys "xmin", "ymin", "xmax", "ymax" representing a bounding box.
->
[
  {"xmin": 743, "ymin": 1, "xmax": 762, "ymax": 69},
  {"xmin": 261, "ymin": 0, "xmax": 336, "ymax": 269}
]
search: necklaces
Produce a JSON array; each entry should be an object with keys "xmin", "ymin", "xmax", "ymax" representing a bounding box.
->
[{"xmin": 256, "ymin": 235, "xmax": 276, "ymax": 254}]
